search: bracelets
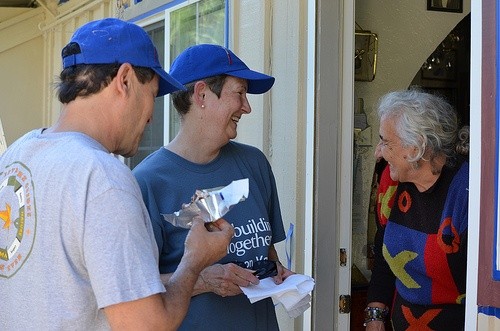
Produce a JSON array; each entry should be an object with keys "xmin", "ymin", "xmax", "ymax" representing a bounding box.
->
[{"xmin": 363, "ymin": 306, "xmax": 389, "ymax": 326}]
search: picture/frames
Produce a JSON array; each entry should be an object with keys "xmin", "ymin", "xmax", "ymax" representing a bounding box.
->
[{"xmin": 427, "ymin": 0, "xmax": 463, "ymax": 13}]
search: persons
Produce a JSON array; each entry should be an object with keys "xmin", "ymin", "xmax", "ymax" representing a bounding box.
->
[
  {"xmin": 0, "ymin": 18, "xmax": 187, "ymax": 331},
  {"xmin": 364, "ymin": 88, "xmax": 470, "ymax": 331},
  {"xmin": 131, "ymin": 44, "xmax": 296, "ymax": 331}
]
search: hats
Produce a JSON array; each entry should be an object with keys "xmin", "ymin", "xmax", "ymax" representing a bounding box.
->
[
  {"xmin": 169, "ymin": 44, "xmax": 275, "ymax": 94},
  {"xmin": 63, "ymin": 17, "xmax": 188, "ymax": 97}
]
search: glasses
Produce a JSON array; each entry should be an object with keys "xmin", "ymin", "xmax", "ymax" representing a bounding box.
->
[{"xmin": 224, "ymin": 260, "xmax": 278, "ymax": 280}]
